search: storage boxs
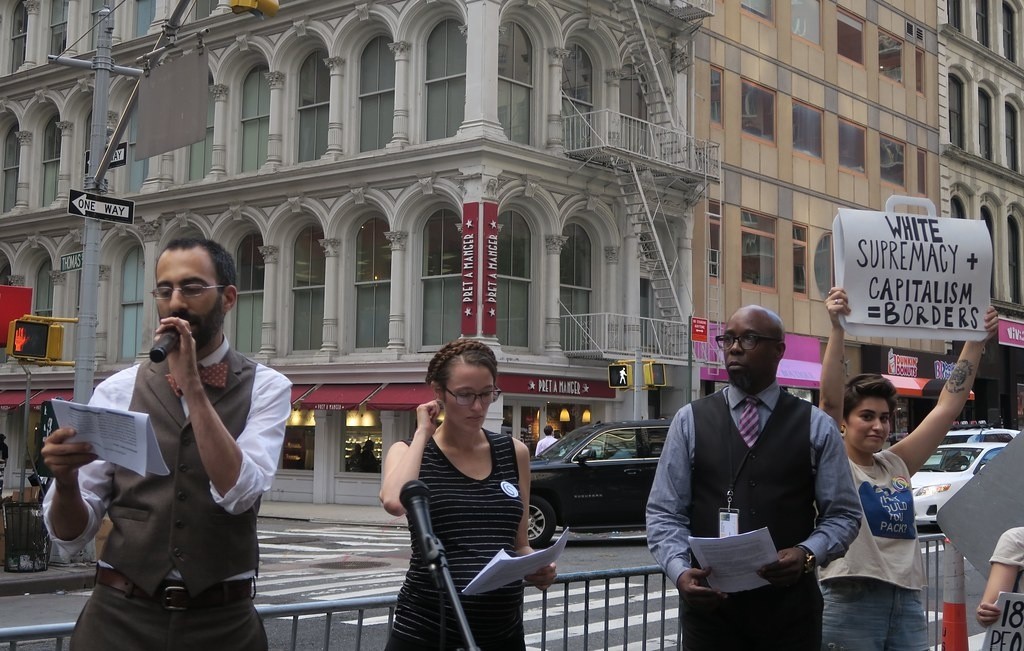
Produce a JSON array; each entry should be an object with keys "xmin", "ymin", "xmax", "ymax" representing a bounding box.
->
[{"xmin": 12, "ymin": 486, "xmax": 40, "ymax": 502}]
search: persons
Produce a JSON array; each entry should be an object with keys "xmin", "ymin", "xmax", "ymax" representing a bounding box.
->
[
  {"xmin": 0, "ymin": 434, "xmax": 9, "ymax": 495},
  {"xmin": 818, "ymin": 287, "xmax": 998, "ymax": 651},
  {"xmin": 609, "ymin": 437, "xmax": 632, "ymax": 458},
  {"xmin": 379, "ymin": 338, "xmax": 557, "ymax": 651},
  {"xmin": 645, "ymin": 304, "xmax": 864, "ymax": 651},
  {"xmin": 41, "ymin": 237, "xmax": 293, "ymax": 651},
  {"xmin": 976, "ymin": 526, "xmax": 1024, "ymax": 628},
  {"xmin": 347, "ymin": 440, "xmax": 378, "ymax": 473},
  {"xmin": 535, "ymin": 426, "xmax": 564, "ymax": 456}
]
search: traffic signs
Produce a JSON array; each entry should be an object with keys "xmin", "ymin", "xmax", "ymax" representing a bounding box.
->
[
  {"xmin": 66, "ymin": 188, "xmax": 136, "ymax": 228},
  {"xmin": 83, "ymin": 140, "xmax": 128, "ymax": 175}
]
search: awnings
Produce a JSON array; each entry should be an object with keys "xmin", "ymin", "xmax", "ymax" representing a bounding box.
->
[
  {"xmin": 290, "ymin": 382, "xmax": 436, "ymax": 412},
  {"xmin": 0, "ymin": 389, "xmax": 74, "ymax": 412},
  {"xmin": 881, "ymin": 374, "xmax": 975, "ymax": 400}
]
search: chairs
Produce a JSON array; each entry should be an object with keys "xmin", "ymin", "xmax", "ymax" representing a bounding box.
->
[{"xmin": 946, "ymin": 456, "xmax": 969, "ymax": 471}]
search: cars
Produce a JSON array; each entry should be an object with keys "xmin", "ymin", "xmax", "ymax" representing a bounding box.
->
[
  {"xmin": 907, "ymin": 441, "xmax": 1009, "ymax": 527},
  {"xmin": 924, "ymin": 418, "xmax": 1024, "ymax": 470},
  {"xmin": 520, "ymin": 417, "xmax": 674, "ymax": 551}
]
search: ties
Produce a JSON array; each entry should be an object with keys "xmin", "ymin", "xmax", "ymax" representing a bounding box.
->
[{"xmin": 738, "ymin": 395, "xmax": 762, "ymax": 448}]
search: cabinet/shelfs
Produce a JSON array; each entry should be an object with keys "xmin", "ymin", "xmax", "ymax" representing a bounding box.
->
[
  {"xmin": 284, "ymin": 446, "xmax": 302, "ymax": 461},
  {"xmin": 345, "ymin": 441, "xmax": 382, "ymax": 459}
]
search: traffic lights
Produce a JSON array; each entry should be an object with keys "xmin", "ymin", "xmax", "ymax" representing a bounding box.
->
[
  {"xmin": 607, "ymin": 364, "xmax": 633, "ymax": 389},
  {"xmin": 7, "ymin": 318, "xmax": 50, "ymax": 360},
  {"xmin": 642, "ymin": 363, "xmax": 667, "ymax": 388}
]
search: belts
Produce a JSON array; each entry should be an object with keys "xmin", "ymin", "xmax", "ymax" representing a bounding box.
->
[{"xmin": 96, "ymin": 566, "xmax": 252, "ymax": 611}]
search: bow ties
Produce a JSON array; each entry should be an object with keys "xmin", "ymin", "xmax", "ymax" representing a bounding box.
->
[{"xmin": 164, "ymin": 363, "xmax": 227, "ymax": 398}]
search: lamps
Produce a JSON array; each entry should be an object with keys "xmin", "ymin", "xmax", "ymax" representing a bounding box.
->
[
  {"xmin": 582, "ymin": 408, "xmax": 591, "ymax": 422},
  {"xmin": 560, "ymin": 408, "xmax": 570, "ymax": 421}
]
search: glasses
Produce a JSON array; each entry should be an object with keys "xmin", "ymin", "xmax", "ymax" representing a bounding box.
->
[
  {"xmin": 716, "ymin": 334, "xmax": 781, "ymax": 351},
  {"xmin": 440, "ymin": 381, "xmax": 501, "ymax": 406},
  {"xmin": 150, "ymin": 284, "xmax": 227, "ymax": 300}
]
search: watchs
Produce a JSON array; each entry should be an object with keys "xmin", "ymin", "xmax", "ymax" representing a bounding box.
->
[{"xmin": 798, "ymin": 546, "xmax": 815, "ymax": 573}]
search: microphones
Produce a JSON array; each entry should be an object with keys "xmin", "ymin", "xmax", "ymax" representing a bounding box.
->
[
  {"xmin": 399, "ymin": 479, "xmax": 444, "ymax": 590},
  {"xmin": 150, "ymin": 329, "xmax": 179, "ymax": 363}
]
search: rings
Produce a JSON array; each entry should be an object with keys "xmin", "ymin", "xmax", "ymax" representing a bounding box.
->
[
  {"xmin": 835, "ymin": 299, "xmax": 838, "ymax": 304},
  {"xmin": 828, "ymin": 292, "xmax": 830, "ymax": 295},
  {"xmin": 978, "ymin": 610, "xmax": 981, "ymax": 614}
]
